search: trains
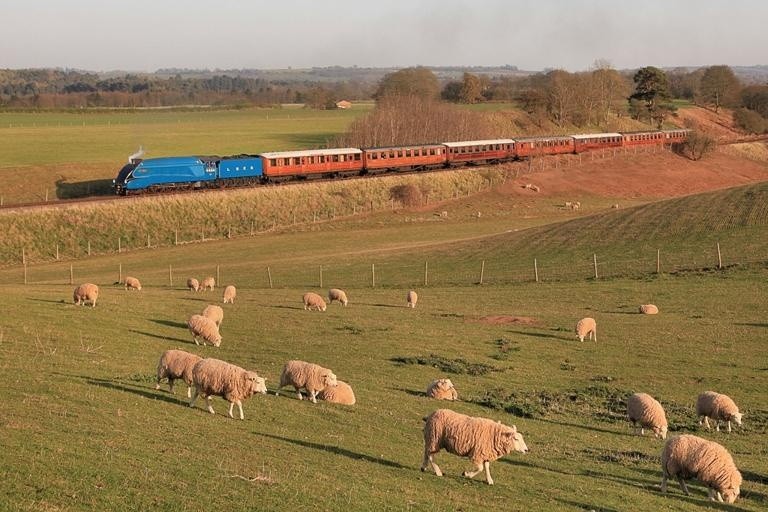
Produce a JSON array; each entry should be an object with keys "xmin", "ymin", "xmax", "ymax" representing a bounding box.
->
[{"xmin": 111, "ymin": 125, "xmax": 699, "ymax": 200}]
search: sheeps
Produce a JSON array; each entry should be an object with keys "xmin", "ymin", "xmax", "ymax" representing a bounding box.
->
[
  {"xmin": 187, "ymin": 277, "xmax": 215, "ymax": 292},
  {"xmin": 124, "ymin": 277, "xmax": 142, "ymax": 292},
  {"xmin": 188, "ymin": 304, "xmax": 224, "ymax": 348},
  {"xmin": 303, "ymin": 292, "xmax": 326, "ymax": 312},
  {"xmin": 661, "ymin": 434, "xmax": 742, "ymax": 504},
  {"xmin": 329, "ymin": 288, "xmax": 348, "ymax": 307},
  {"xmin": 427, "ymin": 378, "xmax": 458, "ymax": 401},
  {"xmin": 73, "ymin": 283, "xmax": 99, "ymax": 308},
  {"xmin": 576, "ymin": 317, "xmax": 597, "ymax": 342},
  {"xmin": 696, "ymin": 390, "xmax": 744, "ymax": 433},
  {"xmin": 628, "ymin": 393, "xmax": 668, "ymax": 440},
  {"xmin": 155, "ymin": 349, "xmax": 268, "ymax": 420},
  {"xmin": 222, "ymin": 285, "xmax": 236, "ymax": 304},
  {"xmin": 640, "ymin": 304, "xmax": 659, "ymax": 314},
  {"xmin": 420, "ymin": 409, "xmax": 528, "ymax": 486},
  {"xmin": 407, "ymin": 291, "xmax": 418, "ymax": 308},
  {"xmin": 276, "ymin": 361, "xmax": 356, "ymax": 406}
]
